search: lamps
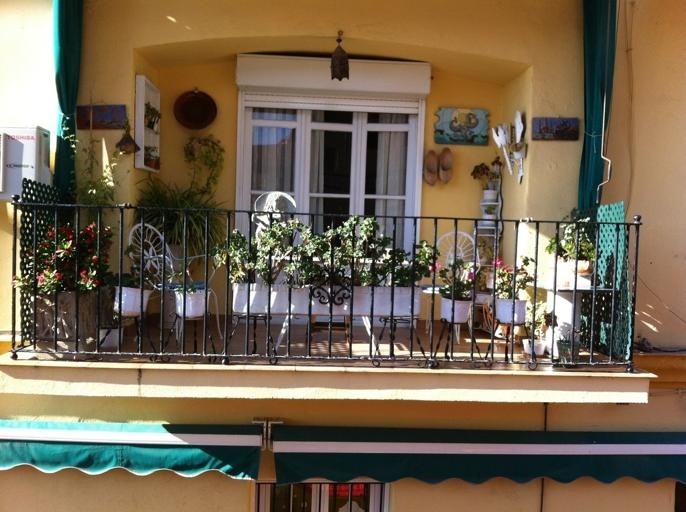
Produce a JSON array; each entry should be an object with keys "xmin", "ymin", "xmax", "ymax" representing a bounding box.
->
[{"xmin": 328, "ymin": 28, "xmax": 350, "ymax": 82}]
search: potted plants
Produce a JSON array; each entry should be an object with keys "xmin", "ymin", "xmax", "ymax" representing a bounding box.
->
[
  {"xmin": 214, "ymin": 217, "xmax": 437, "ymax": 317},
  {"xmin": 110, "ymin": 178, "xmax": 222, "ymax": 332},
  {"xmin": 471, "ymin": 155, "xmax": 504, "ymax": 228},
  {"xmin": 562, "ymin": 217, "xmax": 598, "ymax": 277}
]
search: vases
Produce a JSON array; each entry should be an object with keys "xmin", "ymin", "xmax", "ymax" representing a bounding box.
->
[
  {"xmin": 441, "ymin": 296, "xmax": 529, "ymax": 324},
  {"xmin": 522, "ymin": 338, "xmax": 580, "ymax": 368}
]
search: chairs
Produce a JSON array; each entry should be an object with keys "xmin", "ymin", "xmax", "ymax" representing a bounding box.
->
[{"xmin": 421, "ymin": 231, "xmax": 480, "ymax": 345}]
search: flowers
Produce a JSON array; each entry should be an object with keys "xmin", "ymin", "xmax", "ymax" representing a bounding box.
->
[
  {"xmin": 29, "ymin": 222, "xmax": 112, "ymax": 299},
  {"xmin": 432, "ymin": 256, "xmax": 534, "ymax": 300}
]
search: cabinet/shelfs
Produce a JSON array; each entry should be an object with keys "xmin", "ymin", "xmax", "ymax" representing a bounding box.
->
[{"xmin": 132, "ymin": 74, "xmax": 160, "ymax": 174}]
